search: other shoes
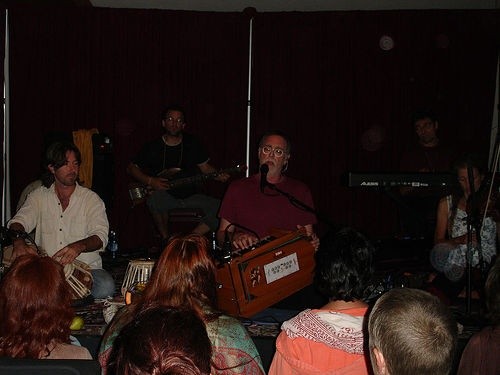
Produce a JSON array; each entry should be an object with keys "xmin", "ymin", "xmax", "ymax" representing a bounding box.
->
[{"xmin": 160, "ymin": 236, "xmax": 169, "ymax": 253}]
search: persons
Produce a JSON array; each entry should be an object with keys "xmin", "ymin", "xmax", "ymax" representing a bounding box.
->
[
  {"xmin": 437, "ymin": 159, "xmax": 500, "ymax": 300},
  {"xmin": 269, "ymin": 255, "xmax": 372, "ymax": 375},
  {"xmin": 398, "ymin": 110, "xmax": 452, "ymax": 232},
  {"xmin": 129, "ymin": 107, "xmax": 231, "ymax": 242},
  {"xmin": 458, "ymin": 258, "xmax": 500, "ymax": 375},
  {"xmin": 217, "ymin": 130, "xmax": 320, "ymax": 320},
  {"xmin": 7, "ymin": 143, "xmax": 114, "ymax": 298},
  {"xmin": 107, "ymin": 304, "xmax": 214, "ymax": 375},
  {"xmin": 0, "ymin": 254, "xmax": 93, "ymax": 360},
  {"xmin": 368, "ymin": 288, "xmax": 457, "ymax": 375},
  {"xmin": 99, "ymin": 232, "xmax": 266, "ymax": 375}
]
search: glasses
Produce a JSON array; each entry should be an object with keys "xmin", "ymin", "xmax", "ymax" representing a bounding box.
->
[
  {"xmin": 167, "ymin": 117, "xmax": 184, "ymax": 125},
  {"xmin": 261, "ymin": 145, "xmax": 288, "ymax": 158}
]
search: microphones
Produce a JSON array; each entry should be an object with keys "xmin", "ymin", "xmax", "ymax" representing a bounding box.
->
[{"xmin": 260, "ymin": 164, "xmax": 269, "ymax": 193}]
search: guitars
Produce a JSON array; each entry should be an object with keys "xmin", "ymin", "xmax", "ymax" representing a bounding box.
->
[{"xmin": 137, "ymin": 158, "xmax": 246, "ymax": 208}]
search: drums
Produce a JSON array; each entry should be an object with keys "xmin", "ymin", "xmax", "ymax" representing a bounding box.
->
[
  {"xmin": 2, "ymin": 246, "xmax": 48, "ymax": 274},
  {"xmin": 53, "ymin": 256, "xmax": 93, "ymax": 299},
  {"xmin": 121, "ymin": 258, "xmax": 158, "ymax": 299}
]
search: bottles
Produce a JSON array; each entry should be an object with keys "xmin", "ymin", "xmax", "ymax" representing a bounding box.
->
[{"xmin": 107, "ymin": 231, "xmax": 119, "ymax": 259}]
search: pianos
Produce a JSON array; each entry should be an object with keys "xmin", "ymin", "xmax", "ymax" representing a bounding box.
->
[{"xmin": 348, "ymin": 170, "xmax": 500, "ymax": 270}]
